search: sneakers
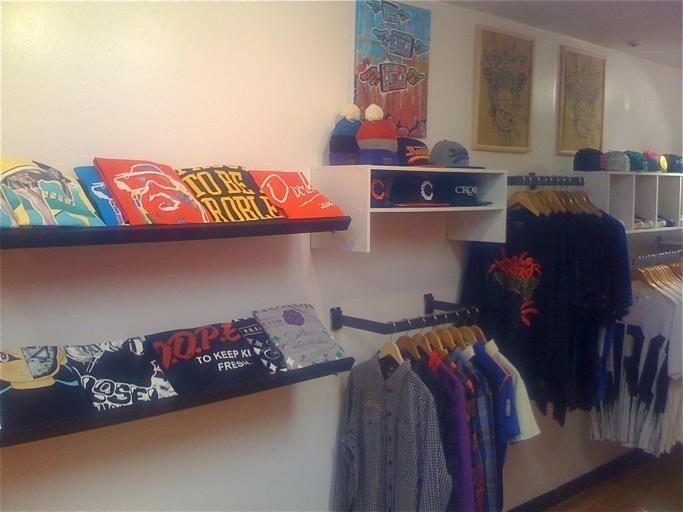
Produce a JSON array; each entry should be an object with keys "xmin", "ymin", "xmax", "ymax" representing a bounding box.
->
[{"xmin": 634, "ymin": 213, "xmax": 675, "ymax": 229}]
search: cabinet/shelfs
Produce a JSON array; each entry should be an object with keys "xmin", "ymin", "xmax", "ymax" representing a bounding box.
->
[
  {"xmin": 569, "ymin": 170, "xmax": 683, "ymax": 235},
  {"xmin": 0, "ymin": 216, "xmax": 355, "ymax": 448},
  {"xmin": 309, "ymin": 165, "xmax": 509, "ymax": 254}
]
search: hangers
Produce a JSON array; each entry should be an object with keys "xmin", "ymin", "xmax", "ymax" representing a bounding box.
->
[
  {"xmin": 506, "ymin": 173, "xmax": 603, "ymax": 218},
  {"xmin": 378, "ymin": 304, "xmax": 488, "ymax": 366},
  {"xmin": 630, "ymin": 246, "xmax": 683, "ymax": 308}
]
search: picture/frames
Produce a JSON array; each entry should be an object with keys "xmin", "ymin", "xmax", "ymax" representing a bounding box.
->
[
  {"xmin": 557, "ymin": 45, "xmax": 607, "ymax": 156},
  {"xmin": 471, "ymin": 24, "xmax": 535, "ymax": 153}
]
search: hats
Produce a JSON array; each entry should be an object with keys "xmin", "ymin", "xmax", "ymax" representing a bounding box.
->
[
  {"xmin": 330, "ymin": 104, "xmax": 363, "ymax": 154},
  {"xmin": 354, "ymin": 103, "xmax": 399, "ymax": 154},
  {"xmin": 574, "ymin": 147, "xmax": 683, "ymax": 173},
  {"xmin": 429, "ymin": 139, "xmax": 486, "ymax": 169},
  {"xmin": 397, "ymin": 137, "xmax": 445, "ymax": 168},
  {"xmin": 371, "ymin": 171, "xmax": 493, "ymax": 208}
]
